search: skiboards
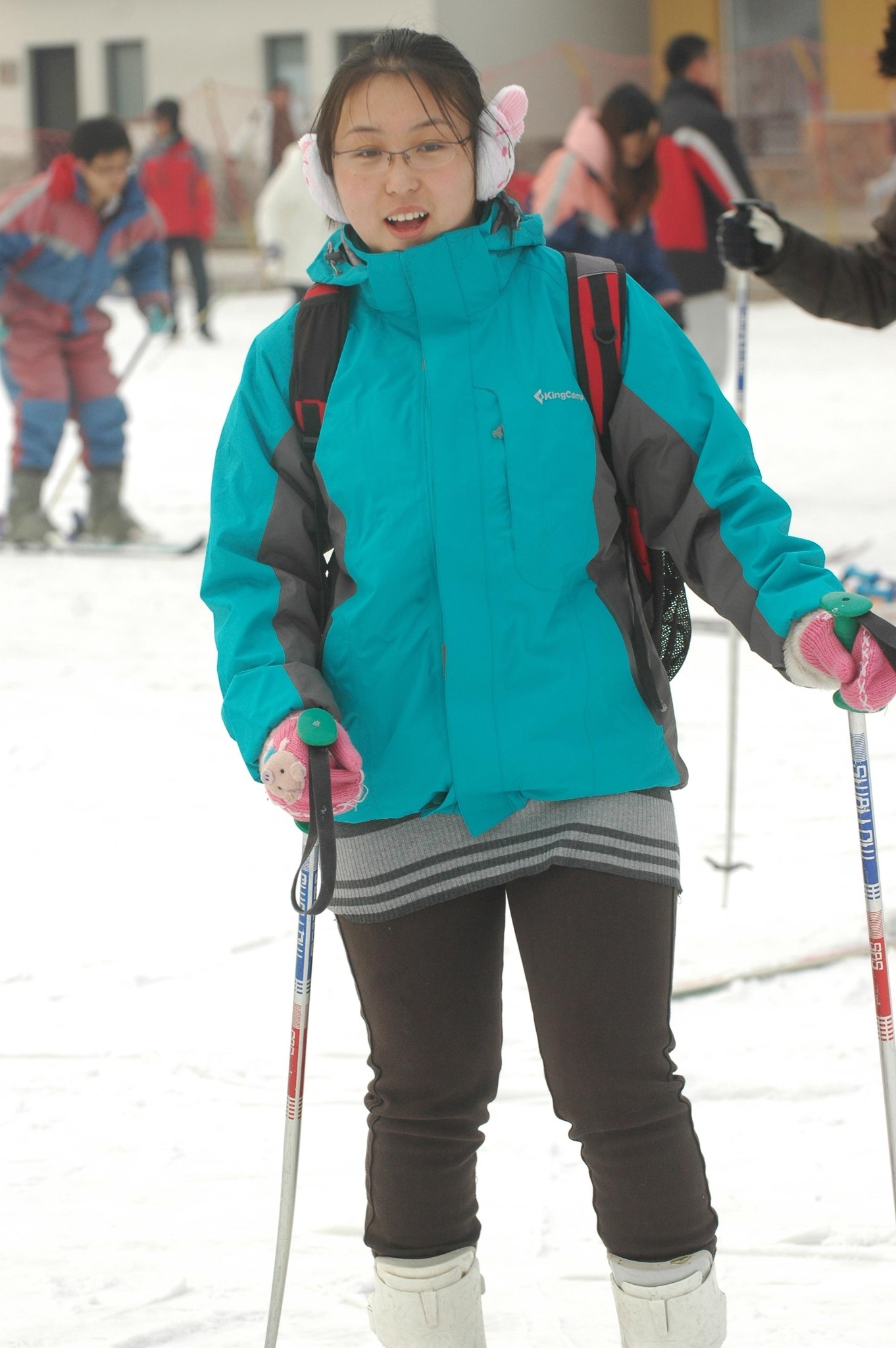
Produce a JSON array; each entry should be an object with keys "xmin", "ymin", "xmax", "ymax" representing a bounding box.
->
[{"xmin": 1, "ymin": 528, "xmax": 208, "ymax": 555}]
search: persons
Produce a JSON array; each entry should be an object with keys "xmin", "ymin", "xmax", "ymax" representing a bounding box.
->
[
  {"xmin": 226, "ymin": 71, "xmax": 309, "ymax": 178},
  {"xmin": 0, "ymin": 117, "xmax": 173, "ymax": 550},
  {"xmin": 524, "ymin": 80, "xmax": 685, "ymax": 335},
  {"xmin": 197, "ymin": 27, "xmax": 896, "ymax": 1348},
  {"xmin": 714, "ymin": 0, "xmax": 896, "ymax": 328},
  {"xmin": 648, "ymin": 32, "xmax": 761, "ymax": 295},
  {"xmin": 134, "ymin": 99, "xmax": 216, "ymax": 341}
]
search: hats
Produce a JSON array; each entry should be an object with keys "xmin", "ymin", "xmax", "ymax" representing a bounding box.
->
[{"xmin": 603, "ymin": 85, "xmax": 661, "ymax": 136}]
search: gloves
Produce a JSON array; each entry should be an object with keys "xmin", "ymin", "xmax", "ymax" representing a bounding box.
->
[
  {"xmin": 258, "ymin": 708, "xmax": 364, "ymax": 822},
  {"xmin": 716, "ymin": 202, "xmax": 783, "ymax": 273},
  {"xmin": 783, "ymin": 608, "xmax": 896, "ymax": 713}
]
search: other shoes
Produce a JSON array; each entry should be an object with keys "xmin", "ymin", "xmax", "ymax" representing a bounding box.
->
[
  {"xmin": 90, "ymin": 509, "xmax": 146, "ymax": 540},
  {"xmin": 4, "ymin": 512, "xmax": 54, "ymax": 545}
]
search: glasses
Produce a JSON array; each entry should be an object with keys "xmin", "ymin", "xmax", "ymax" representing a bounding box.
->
[
  {"xmin": 86, "ymin": 161, "xmax": 140, "ymax": 177},
  {"xmin": 330, "ymin": 136, "xmax": 476, "ymax": 176}
]
